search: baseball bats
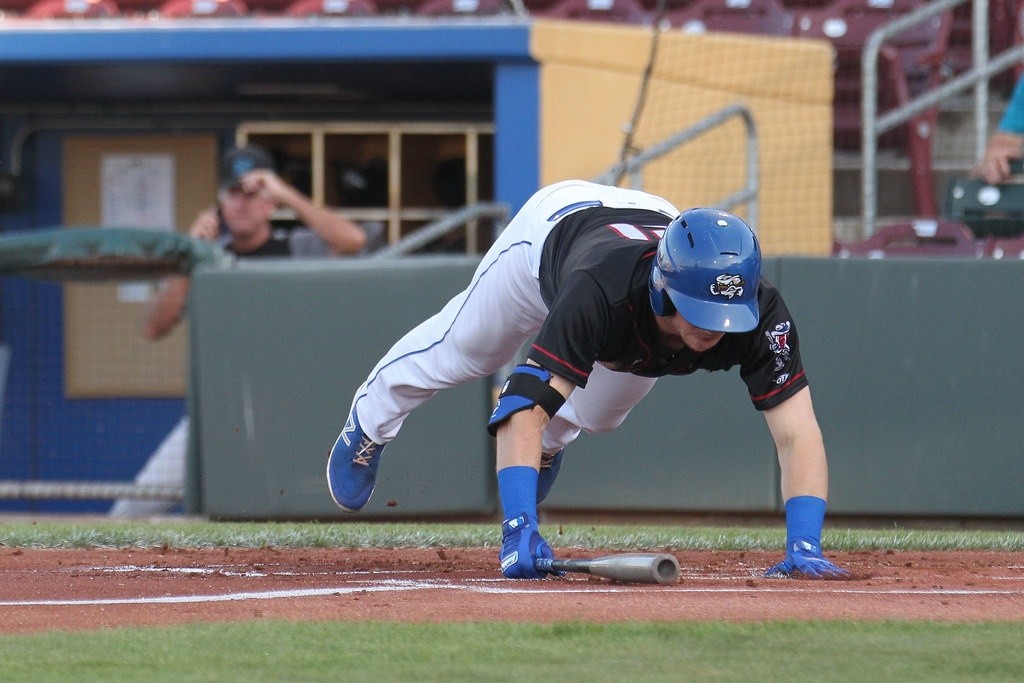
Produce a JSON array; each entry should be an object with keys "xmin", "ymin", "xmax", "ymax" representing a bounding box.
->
[{"xmin": 535, "ymin": 553, "xmax": 680, "ymax": 584}]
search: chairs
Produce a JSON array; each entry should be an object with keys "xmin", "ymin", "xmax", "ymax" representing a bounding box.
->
[
  {"xmin": 802, "ymin": 1, "xmax": 1023, "ymax": 259},
  {"xmin": 0, "ymin": 0, "xmax": 789, "ymax": 37}
]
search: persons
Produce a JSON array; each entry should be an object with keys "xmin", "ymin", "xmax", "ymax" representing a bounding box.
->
[
  {"xmin": 326, "ymin": 176, "xmax": 846, "ymax": 584},
  {"xmin": 144, "ymin": 143, "xmax": 366, "ymax": 345}
]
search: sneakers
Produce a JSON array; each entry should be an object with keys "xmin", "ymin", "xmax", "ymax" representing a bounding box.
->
[
  {"xmin": 535, "ymin": 445, "xmax": 565, "ymax": 505},
  {"xmin": 326, "ymin": 382, "xmax": 390, "ymax": 513}
]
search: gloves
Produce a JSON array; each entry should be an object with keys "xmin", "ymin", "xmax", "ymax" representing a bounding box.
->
[
  {"xmin": 497, "ymin": 465, "xmax": 562, "ymax": 579},
  {"xmin": 764, "ymin": 496, "xmax": 852, "ymax": 579}
]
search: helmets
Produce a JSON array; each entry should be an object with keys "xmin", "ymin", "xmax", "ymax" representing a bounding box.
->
[{"xmin": 656, "ymin": 207, "xmax": 763, "ymax": 332}]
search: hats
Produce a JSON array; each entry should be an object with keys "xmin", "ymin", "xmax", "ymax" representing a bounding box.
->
[{"xmin": 217, "ymin": 142, "xmax": 278, "ymax": 187}]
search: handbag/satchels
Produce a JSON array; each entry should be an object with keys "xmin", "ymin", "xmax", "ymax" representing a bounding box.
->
[{"xmin": 949, "ymin": 165, "xmax": 1023, "ymax": 224}]
